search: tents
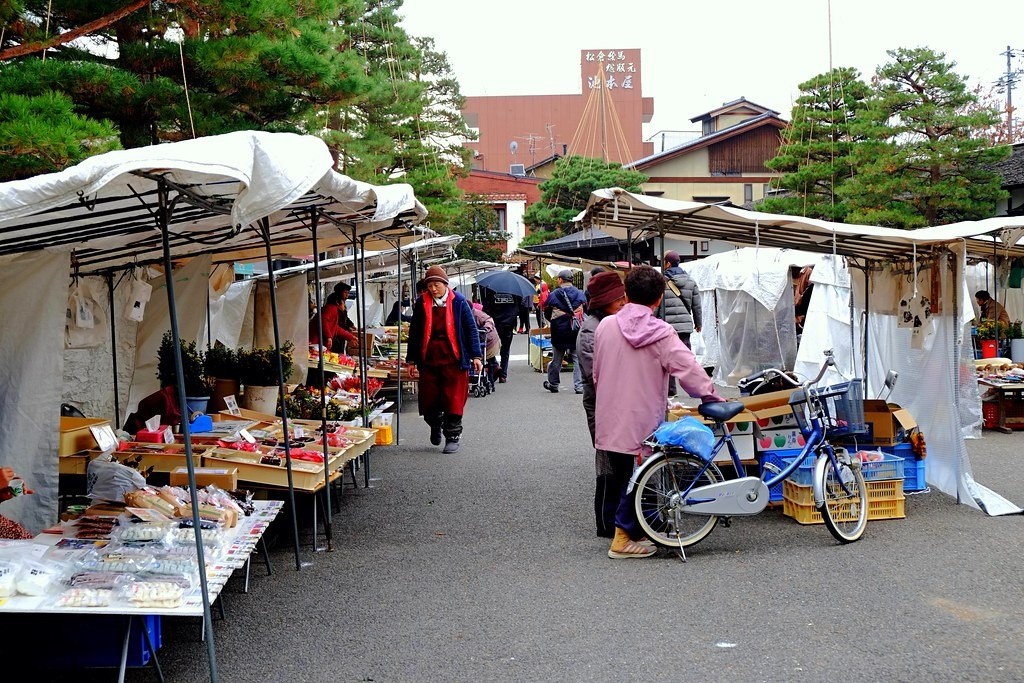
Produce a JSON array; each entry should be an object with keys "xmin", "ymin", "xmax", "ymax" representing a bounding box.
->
[
  {"xmin": 517, "ymin": 185, "xmax": 1024, "ymax": 514},
  {"xmin": 0, "ymin": 128, "xmax": 506, "ymax": 683}
]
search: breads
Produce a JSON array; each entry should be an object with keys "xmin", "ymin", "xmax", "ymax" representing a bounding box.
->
[{"xmin": 131, "ymin": 489, "xmax": 239, "ymax": 529}]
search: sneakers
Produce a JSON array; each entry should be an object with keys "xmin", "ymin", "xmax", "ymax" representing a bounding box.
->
[{"xmin": 543, "ymin": 381, "xmax": 559, "ymax": 393}]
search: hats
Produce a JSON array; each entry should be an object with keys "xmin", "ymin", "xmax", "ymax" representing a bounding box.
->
[
  {"xmin": 467, "ymin": 300, "xmax": 473, "ymax": 309},
  {"xmin": 558, "ymin": 270, "xmax": 574, "ymax": 280},
  {"xmin": 657, "ymin": 250, "xmax": 680, "ymax": 263},
  {"xmin": 591, "ymin": 268, "xmax": 605, "ymax": 276},
  {"xmin": 587, "ymin": 270, "xmax": 626, "ymax": 310},
  {"xmin": 424, "ymin": 266, "xmax": 449, "ymax": 285},
  {"xmin": 334, "ymin": 281, "xmax": 352, "ymax": 293},
  {"xmin": 534, "ymin": 270, "xmax": 543, "ymax": 280}
]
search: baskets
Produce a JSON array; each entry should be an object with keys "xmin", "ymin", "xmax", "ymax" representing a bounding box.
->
[
  {"xmin": 982, "ymin": 399, "xmax": 1024, "ymax": 429},
  {"xmin": 788, "ymin": 378, "xmax": 866, "ymax": 440},
  {"xmin": 379, "ymin": 413, "xmax": 394, "ymax": 426}
]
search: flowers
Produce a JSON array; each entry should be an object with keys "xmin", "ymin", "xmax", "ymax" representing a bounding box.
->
[
  {"xmin": 156, "ymin": 331, "xmax": 387, "ymax": 422},
  {"xmin": 972, "ymin": 316, "xmax": 1024, "ymax": 341}
]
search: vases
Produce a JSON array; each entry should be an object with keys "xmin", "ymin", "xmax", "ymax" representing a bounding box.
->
[
  {"xmin": 244, "ymin": 385, "xmax": 279, "ymax": 416},
  {"xmin": 214, "ymin": 378, "xmax": 240, "ymax": 411},
  {"xmin": 1010, "ymin": 338, "xmax": 1024, "ymax": 362},
  {"xmin": 184, "ymin": 396, "xmax": 211, "ymax": 419},
  {"xmin": 980, "ymin": 340, "xmax": 999, "ymax": 359}
]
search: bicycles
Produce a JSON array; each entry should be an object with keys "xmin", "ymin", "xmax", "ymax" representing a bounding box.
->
[{"xmin": 624, "ymin": 343, "xmax": 867, "ymax": 564}]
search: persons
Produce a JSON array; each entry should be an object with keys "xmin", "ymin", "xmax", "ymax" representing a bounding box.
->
[
  {"xmin": 794, "ymin": 267, "xmax": 814, "ymax": 333},
  {"xmin": 975, "ymin": 290, "xmax": 1010, "ymax": 327},
  {"xmin": 309, "ymin": 250, "xmax": 725, "ymax": 559}
]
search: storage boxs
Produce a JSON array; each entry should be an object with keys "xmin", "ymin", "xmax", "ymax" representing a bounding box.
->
[
  {"xmin": 982, "ymin": 399, "xmax": 1024, "ymax": 430},
  {"xmin": 348, "ymin": 348, "xmax": 374, "ymax": 356},
  {"xmin": 531, "ymin": 336, "xmax": 550, "ymax": 347},
  {"xmin": 40, "ymin": 613, "xmax": 162, "ymax": 668},
  {"xmin": 348, "ymin": 333, "xmax": 375, "ymax": 348},
  {"xmin": 60, "ymin": 413, "xmax": 395, "ymax": 491},
  {"xmin": 664, "ymin": 372, "xmax": 927, "ymax": 525},
  {"xmin": 973, "ymin": 348, "xmax": 1005, "ymax": 359}
]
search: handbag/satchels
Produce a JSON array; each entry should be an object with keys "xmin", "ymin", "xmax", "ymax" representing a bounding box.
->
[
  {"xmin": 86, "ymin": 447, "xmax": 147, "ymax": 505},
  {"xmin": 654, "ymin": 415, "xmax": 715, "ymax": 460},
  {"xmin": 695, "ymin": 331, "xmax": 707, "ymax": 365},
  {"xmin": 805, "ymin": 385, "xmax": 837, "ymax": 431},
  {"xmin": 563, "ymin": 289, "xmax": 588, "ymax": 331}
]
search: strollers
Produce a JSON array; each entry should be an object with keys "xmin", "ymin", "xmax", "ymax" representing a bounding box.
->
[{"xmin": 468, "ymin": 327, "xmax": 491, "ymax": 397}]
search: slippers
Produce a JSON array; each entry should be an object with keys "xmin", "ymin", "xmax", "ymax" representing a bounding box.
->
[{"xmin": 608, "ymin": 539, "xmax": 657, "ymax": 559}]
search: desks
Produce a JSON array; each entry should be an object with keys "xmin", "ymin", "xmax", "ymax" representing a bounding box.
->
[
  {"xmin": 972, "ymin": 335, "xmax": 979, "ymax": 359},
  {"xmin": 977, "ymin": 378, "xmax": 1024, "ymax": 434},
  {"xmin": 0, "ymin": 446, "xmax": 378, "ymax": 683},
  {"xmin": 365, "ymin": 400, "xmax": 395, "ymax": 424},
  {"xmin": 307, "ymin": 324, "xmax": 418, "ymax": 408},
  {"xmin": 528, "ymin": 327, "xmax": 574, "ymax": 374}
]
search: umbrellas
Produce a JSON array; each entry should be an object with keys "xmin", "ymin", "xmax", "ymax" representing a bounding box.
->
[{"xmin": 474, "ymin": 270, "xmax": 537, "ymax": 296}]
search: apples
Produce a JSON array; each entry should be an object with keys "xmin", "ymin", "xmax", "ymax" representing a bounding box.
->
[
  {"xmin": 727, "ymin": 415, "xmax": 805, "ymax": 448},
  {"xmin": 857, "ymin": 451, "xmax": 881, "ymax": 462}
]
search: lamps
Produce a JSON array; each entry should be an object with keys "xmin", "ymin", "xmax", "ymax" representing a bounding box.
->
[{"xmin": 701, "ymin": 241, "xmax": 708, "ymax": 251}]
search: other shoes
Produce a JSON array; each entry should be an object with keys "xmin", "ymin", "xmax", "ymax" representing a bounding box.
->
[
  {"xmin": 442, "ymin": 441, "xmax": 459, "ymax": 452},
  {"xmin": 492, "ymin": 364, "xmax": 506, "ymax": 383},
  {"xmin": 512, "ymin": 328, "xmax": 529, "ymax": 334},
  {"xmin": 430, "ymin": 422, "xmax": 441, "ymax": 445}
]
